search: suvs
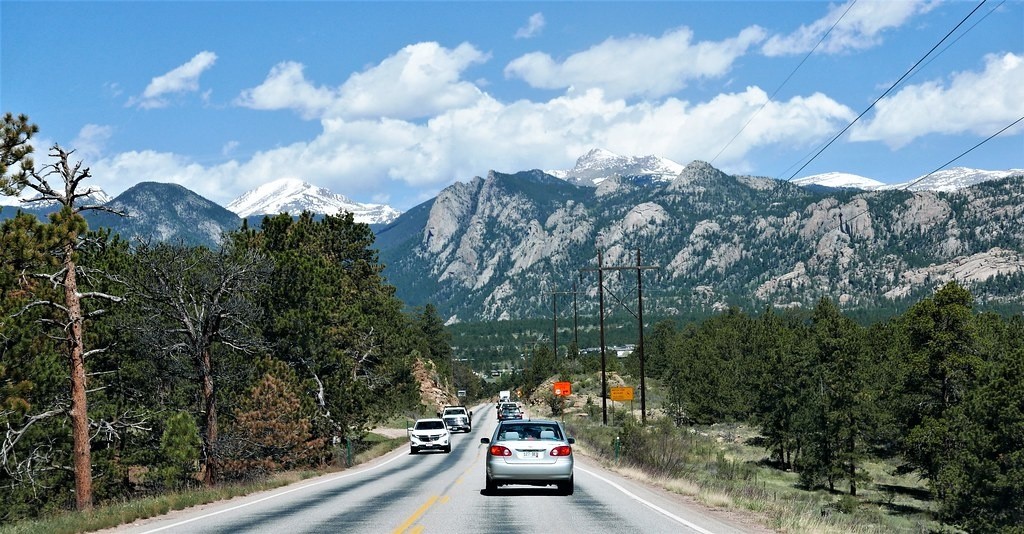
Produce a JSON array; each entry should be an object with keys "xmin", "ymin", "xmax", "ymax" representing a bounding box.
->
[
  {"xmin": 437, "ymin": 405, "xmax": 474, "ymax": 433},
  {"xmin": 408, "ymin": 418, "xmax": 453, "ymax": 454}
]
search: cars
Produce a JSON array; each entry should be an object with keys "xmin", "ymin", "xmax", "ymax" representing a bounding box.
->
[
  {"xmin": 479, "ymin": 417, "xmax": 577, "ymax": 496},
  {"xmin": 496, "ymin": 390, "xmax": 524, "ymax": 423}
]
